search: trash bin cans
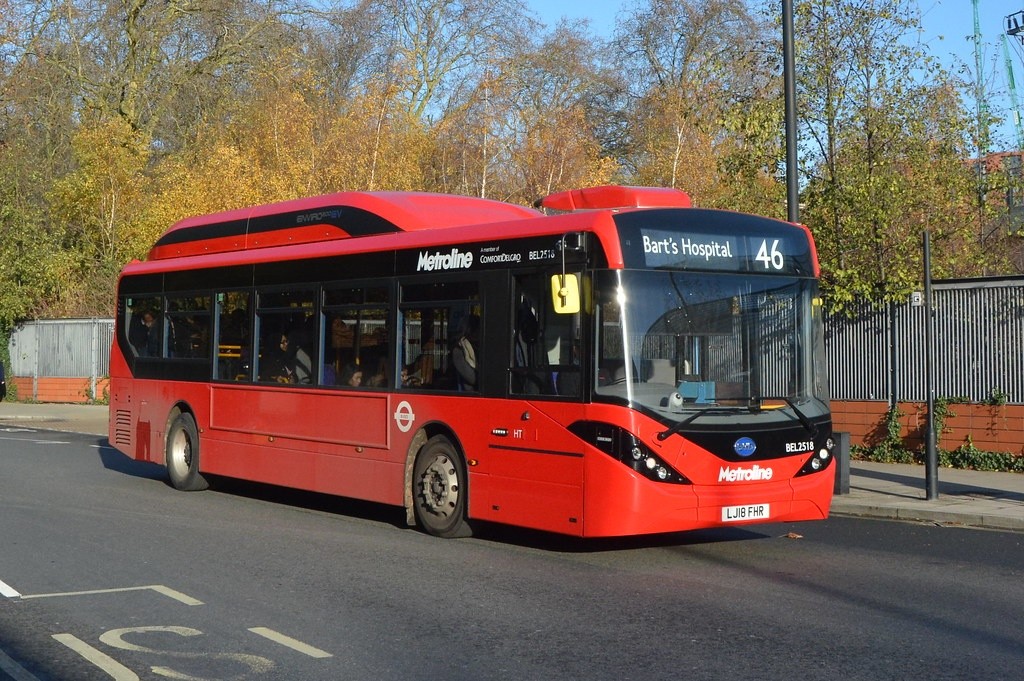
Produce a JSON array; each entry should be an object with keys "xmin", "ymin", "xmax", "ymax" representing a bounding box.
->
[{"xmin": 832, "ymin": 432, "xmax": 850, "ymax": 494}]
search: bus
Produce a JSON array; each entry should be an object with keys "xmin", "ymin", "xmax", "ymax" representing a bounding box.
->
[{"xmin": 106, "ymin": 185, "xmax": 837, "ymax": 539}]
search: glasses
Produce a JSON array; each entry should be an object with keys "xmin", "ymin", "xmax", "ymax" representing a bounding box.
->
[{"xmin": 280, "ymin": 339, "xmax": 290, "ymax": 345}]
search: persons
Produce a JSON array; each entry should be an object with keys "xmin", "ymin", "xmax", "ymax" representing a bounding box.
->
[{"xmin": 133, "ymin": 293, "xmax": 578, "ymax": 392}]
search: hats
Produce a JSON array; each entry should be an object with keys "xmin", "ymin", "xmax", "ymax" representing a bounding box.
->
[{"xmin": 344, "ymin": 356, "xmax": 362, "ymax": 382}]
